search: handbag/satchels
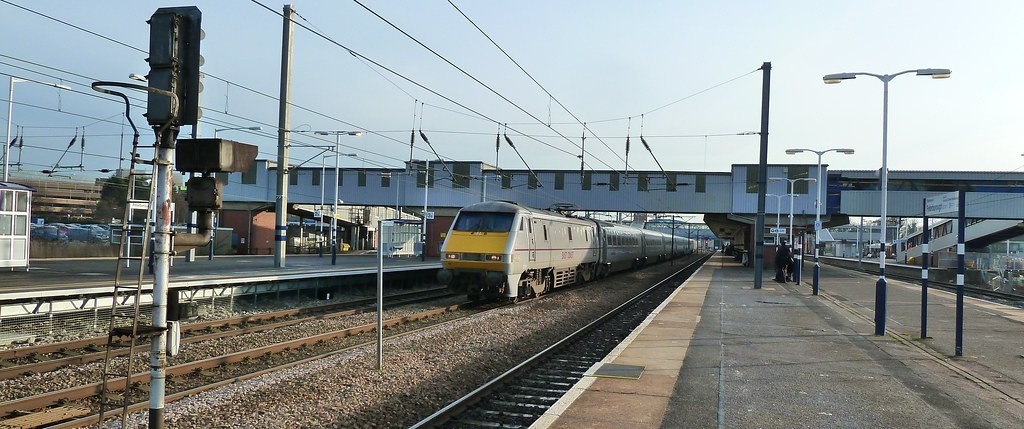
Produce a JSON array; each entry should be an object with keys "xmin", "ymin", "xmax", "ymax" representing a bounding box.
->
[{"xmin": 786, "ymin": 257, "xmax": 794, "ymax": 265}]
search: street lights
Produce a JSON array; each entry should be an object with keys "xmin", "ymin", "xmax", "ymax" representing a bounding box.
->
[
  {"xmin": 314, "ymin": 130, "xmax": 363, "ymax": 263},
  {"xmin": 765, "ymin": 193, "xmax": 799, "ymax": 281},
  {"xmin": 768, "ymin": 177, "xmax": 817, "ymax": 287},
  {"xmin": 785, "ymin": 148, "xmax": 854, "ymax": 297},
  {"xmin": 212, "ymin": 125, "xmax": 262, "ymax": 137},
  {"xmin": 3, "ymin": 73, "xmax": 73, "ymax": 184},
  {"xmin": 823, "ymin": 68, "xmax": 952, "ymax": 338}
]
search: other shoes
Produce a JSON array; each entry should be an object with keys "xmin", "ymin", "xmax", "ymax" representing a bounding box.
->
[{"xmin": 777, "ymin": 280, "xmax": 785, "ymax": 283}]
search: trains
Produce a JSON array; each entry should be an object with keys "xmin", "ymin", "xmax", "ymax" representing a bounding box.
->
[{"xmin": 441, "ymin": 198, "xmax": 695, "ymax": 304}]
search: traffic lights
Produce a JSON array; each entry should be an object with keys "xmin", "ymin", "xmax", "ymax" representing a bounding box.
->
[{"xmin": 153, "ymin": 6, "xmax": 205, "ymax": 126}]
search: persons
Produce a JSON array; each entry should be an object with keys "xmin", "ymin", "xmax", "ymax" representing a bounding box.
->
[
  {"xmin": 726, "ymin": 244, "xmax": 728, "ymax": 247},
  {"xmin": 772, "ymin": 241, "xmax": 791, "ymax": 283},
  {"xmin": 722, "ymin": 244, "xmax": 724, "ymax": 250}
]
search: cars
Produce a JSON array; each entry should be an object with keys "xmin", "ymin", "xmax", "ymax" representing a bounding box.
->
[{"xmin": 29, "ymin": 222, "xmax": 111, "ymax": 244}]
